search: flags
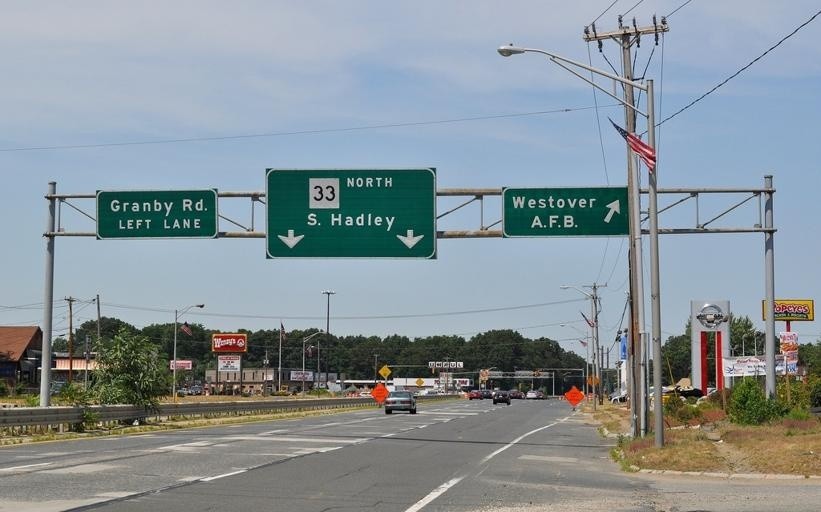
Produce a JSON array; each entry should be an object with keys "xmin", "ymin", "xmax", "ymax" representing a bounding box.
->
[{"xmin": 608, "ymin": 116, "xmax": 656, "ymax": 171}]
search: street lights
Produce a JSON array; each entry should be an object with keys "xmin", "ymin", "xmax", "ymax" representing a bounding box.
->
[
  {"xmin": 730, "ymin": 329, "xmax": 761, "ymax": 389},
  {"xmin": 302, "ymin": 331, "xmax": 327, "ymax": 375},
  {"xmin": 496, "ymin": 43, "xmax": 666, "ymax": 449},
  {"xmin": 484, "ymin": 367, "xmax": 497, "ymax": 390},
  {"xmin": 372, "ymin": 354, "xmax": 380, "ymax": 387},
  {"xmin": 171, "ymin": 303, "xmax": 205, "ymax": 403},
  {"xmin": 559, "ymin": 323, "xmax": 589, "ymax": 396},
  {"xmin": 321, "ymin": 289, "xmax": 336, "ymax": 389},
  {"xmin": 558, "ymin": 284, "xmax": 605, "ymax": 406},
  {"xmin": 91, "ymin": 293, "xmax": 101, "ymax": 339}
]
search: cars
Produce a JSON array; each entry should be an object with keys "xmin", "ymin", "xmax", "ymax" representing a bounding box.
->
[
  {"xmin": 605, "ymin": 384, "xmax": 706, "ymax": 406},
  {"xmin": 383, "ymin": 390, "xmax": 417, "ymax": 415},
  {"xmin": 186, "ymin": 383, "xmax": 373, "ymax": 398},
  {"xmin": 468, "ymin": 389, "xmax": 548, "ymax": 405}
]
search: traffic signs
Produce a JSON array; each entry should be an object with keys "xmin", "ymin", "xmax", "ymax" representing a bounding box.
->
[
  {"xmin": 265, "ymin": 167, "xmax": 436, "ymax": 258},
  {"xmin": 501, "ymin": 186, "xmax": 630, "ymax": 236},
  {"xmin": 96, "ymin": 189, "xmax": 218, "ymax": 238}
]
